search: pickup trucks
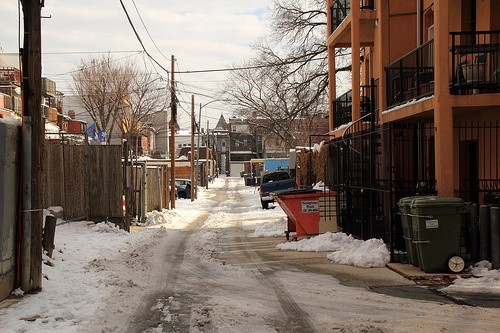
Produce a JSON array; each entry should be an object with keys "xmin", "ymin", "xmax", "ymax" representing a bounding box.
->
[{"xmin": 258, "ymin": 169, "xmax": 296, "ymax": 209}]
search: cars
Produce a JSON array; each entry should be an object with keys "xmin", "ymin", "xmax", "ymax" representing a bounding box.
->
[{"xmin": 167, "ymin": 178, "xmax": 191, "ymax": 199}]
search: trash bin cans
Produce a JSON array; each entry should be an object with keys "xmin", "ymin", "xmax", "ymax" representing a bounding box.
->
[
  {"xmin": 267, "ymin": 188, "xmax": 345, "ymax": 240},
  {"xmin": 394, "ymin": 193, "xmax": 466, "ymax": 276}
]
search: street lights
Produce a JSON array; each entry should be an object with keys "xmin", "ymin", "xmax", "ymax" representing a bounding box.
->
[{"xmin": 194, "ymin": 98, "xmax": 232, "ymax": 200}]
